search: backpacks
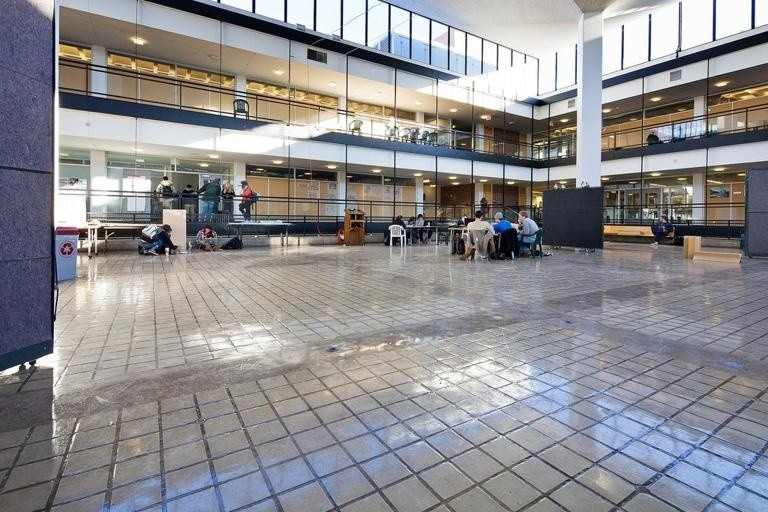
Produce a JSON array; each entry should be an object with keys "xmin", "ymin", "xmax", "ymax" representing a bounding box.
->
[
  {"xmin": 142, "ymin": 225, "xmax": 165, "ymax": 239},
  {"xmin": 251, "ymin": 191, "xmax": 259, "ymax": 202},
  {"xmin": 163, "ymin": 186, "xmax": 171, "ymax": 196},
  {"xmin": 452, "ymin": 237, "xmax": 465, "ymax": 254}
]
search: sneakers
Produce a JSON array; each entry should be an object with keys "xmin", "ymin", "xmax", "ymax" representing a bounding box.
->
[{"xmin": 144, "ymin": 249, "xmax": 159, "ymax": 256}]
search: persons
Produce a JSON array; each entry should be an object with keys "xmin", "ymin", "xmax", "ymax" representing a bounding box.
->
[
  {"xmin": 137, "ymin": 176, "xmax": 253, "ymax": 256},
  {"xmin": 650, "ymin": 215, "xmax": 673, "ymax": 246},
  {"xmin": 385, "ymin": 214, "xmax": 425, "ymax": 245},
  {"xmin": 451, "ymin": 197, "xmax": 540, "ymax": 263}
]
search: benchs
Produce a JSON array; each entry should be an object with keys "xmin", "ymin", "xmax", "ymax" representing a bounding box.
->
[{"xmin": 603, "ymin": 225, "xmax": 676, "ymax": 244}]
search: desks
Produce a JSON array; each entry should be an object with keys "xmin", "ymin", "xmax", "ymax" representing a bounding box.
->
[
  {"xmin": 226, "ymin": 222, "xmax": 293, "ymax": 248},
  {"xmin": 87, "ymin": 222, "xmax": 164, "ymax": 260}
]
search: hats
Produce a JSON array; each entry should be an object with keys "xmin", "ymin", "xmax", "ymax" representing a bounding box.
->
[{"xmin": 495, "ymin": 213, "xmax": 503, "ymax": 218}]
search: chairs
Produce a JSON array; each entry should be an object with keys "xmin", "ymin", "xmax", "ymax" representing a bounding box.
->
[
  {"xmin": 232, "ymin": 98, "xmax": 250, "ymax": 121},
  {"xmin": 349, "ymin": 119, "xmax": 364, "ymax": 135},
  {"xmin": 383, "ymin": 217, "xmax": 545, "ymax": 261},
  {"xmin": 384, "ymin": 125, "xmax": 438, "ymax": 147},
  {"xmin": 646, "ymin": 134, "xmax": 663, "ymax": 145}
]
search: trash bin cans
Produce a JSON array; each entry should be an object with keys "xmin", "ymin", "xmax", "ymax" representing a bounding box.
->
[{"xmin": 53, "ymin": 226, "xmax": 81, "ymax": 283}]
